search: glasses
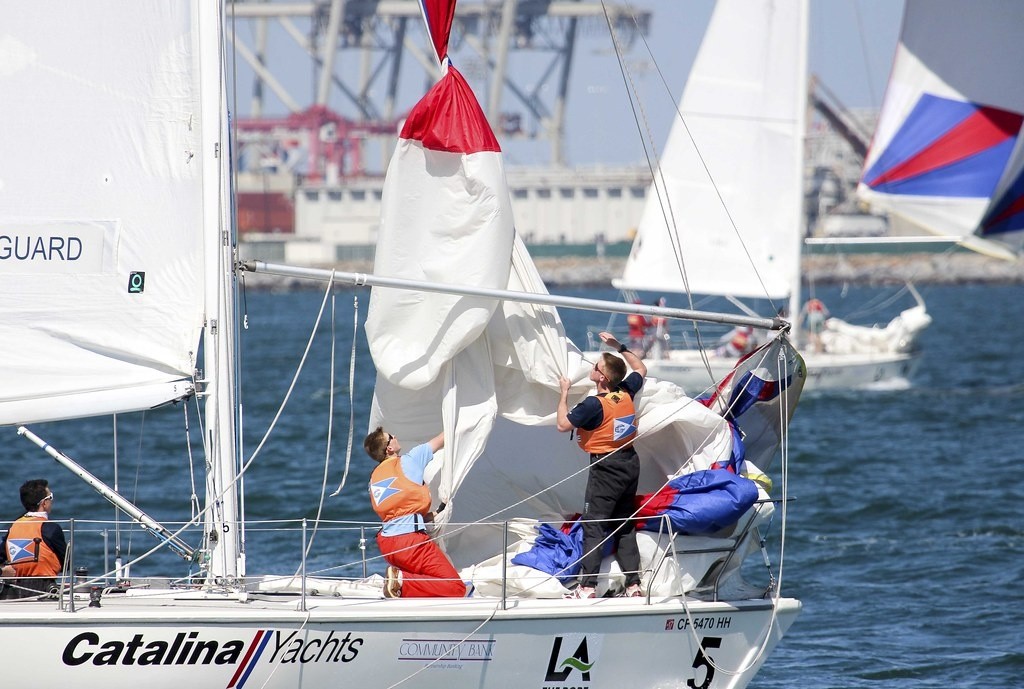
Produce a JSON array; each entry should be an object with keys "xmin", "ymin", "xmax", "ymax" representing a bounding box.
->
[
  {"xmin": 384, "ymin": 432, "xmax": 394, "ymax": 455},
  {"xmin": 37, "ymin": 492, "xmax": 54, "ymax": 505},
  {"xmin": 594, "ymin": 363, "xmax": 611, "ymax": 382}
]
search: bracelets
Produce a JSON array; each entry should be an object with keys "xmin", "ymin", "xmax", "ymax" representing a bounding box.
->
[{"xmin": 617, "ymin": 344, "xmax": 627, "ymax": 353}]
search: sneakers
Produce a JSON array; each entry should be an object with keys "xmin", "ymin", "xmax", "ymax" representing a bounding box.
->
[
  {"xmin": 383, "ymin": 565, "xmax": 402, "ymax": 598},
  {"xmin": 561, "ymin": 583, "xmax": 597, "ymax": 598},
  {"xmin": 615, "ymin": 583, "xmax": 641, "ymax": 597}
]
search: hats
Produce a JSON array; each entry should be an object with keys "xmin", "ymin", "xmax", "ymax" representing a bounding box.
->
[{"xmin": 633, "ymin": 300, "xmax": 641, "ymax": 304}]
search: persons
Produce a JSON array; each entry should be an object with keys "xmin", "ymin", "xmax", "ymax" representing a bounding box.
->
[
  {"xmin": 716, "ymin": 325, "xmax": 757, "ymax": 359},
  {"xmin": 626, "ymin": 299, "xmax": 667, "ymax": 359},
  {"xmin": 364, "ymin": 426, "xmax": 467, "ymax": 598},
  {"xmin": 801, "ymin": 295, "xmax": 831, "ymax": 352},
  {"xmin": 558, "ymin": 330, "xmax": 647, "ymax": 598},
  {"xmin": 0, "ymin": 479, "xmax": 70, "ymax": 600}
]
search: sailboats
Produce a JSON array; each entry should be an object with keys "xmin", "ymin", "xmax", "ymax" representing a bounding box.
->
[
  {"xmin": 803, "ymin": 0, "xmax": 1024, "ymax": 280},
  {"xmin": 568, "ymin": 0, "xmax": 934, "ymax": 396},
  {"xmin": 0, "ymin": 0, "xmax": 802, "ymax": 686}
]
